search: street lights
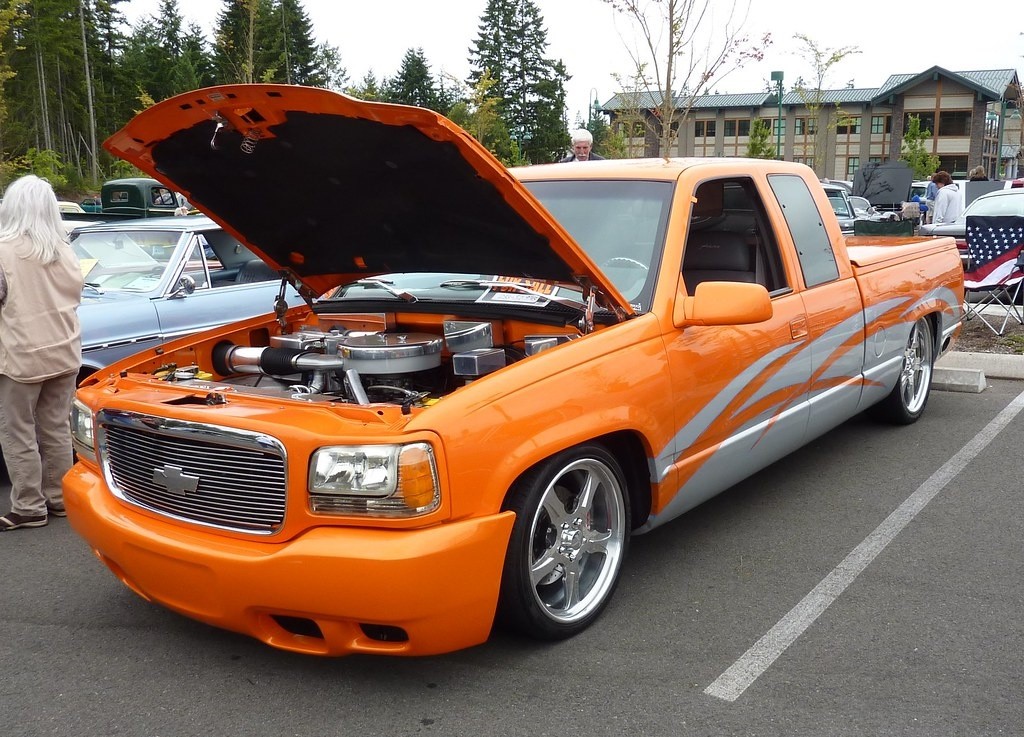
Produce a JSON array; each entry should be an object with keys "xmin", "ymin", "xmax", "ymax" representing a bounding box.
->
[
  {"xmin": 589, "ymin": 87, "xmax": 605, "ymax": 133},
  {"xmin": 510, "ymin": 124, "xmax": 531, "ymax": 166},
  {"xmin": 771, "ymin": 71, "xmax": 784, "ymax": 160},
  {"xmin": 987, "ymin": 100, "xmax": 1022, "ymax": 180}
]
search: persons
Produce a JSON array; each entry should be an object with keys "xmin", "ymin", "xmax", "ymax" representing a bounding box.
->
[
  {"xmin": 925, "ymin": 164, "xmax": 989, "ymax": 223},
  {"xmin": 0, "ymin": 170, "xmax": 82, "ymax": 530},
  {"xmin": 559, "ymin": 128, "xmax": 606, "ymax": 162}
]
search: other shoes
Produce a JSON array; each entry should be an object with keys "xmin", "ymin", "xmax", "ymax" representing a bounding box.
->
[
  {"xmin": 46, "ymin": 505, "xmax": 66, "ymax": 517},
  {"xmin": 0, "ymin": 512, "xmax": 49, "ymax": 531}
]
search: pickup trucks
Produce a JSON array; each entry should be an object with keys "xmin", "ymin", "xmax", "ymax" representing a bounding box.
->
[{"xmin": 61, "ymin": 85, "xmax": 964, "ymax": 657}]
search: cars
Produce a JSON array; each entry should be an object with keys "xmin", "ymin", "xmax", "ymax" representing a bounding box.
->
[
  {"xmin": 721, "ymin": 165, "xmax": 1024, "ymax": 237},
  {"xmin": 58, "ymin": 178, "xmax": 325, "ymax": 387}
]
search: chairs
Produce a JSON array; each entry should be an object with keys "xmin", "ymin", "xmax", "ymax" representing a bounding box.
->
[
  {"xmin": 682, "ymin": 229, "xmax": 755, "ymax": 296},
  {"xmin": 963, "ymin": 215, "xmax": 1024, "ymax": 336}
]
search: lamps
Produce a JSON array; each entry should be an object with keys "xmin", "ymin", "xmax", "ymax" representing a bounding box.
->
[
  {"xmin": 987, "ymin": 101, "xmax": 997, "ymax": 120},
  {"xmin": 1010, "ymin": 96, "xmax": 1022, "ymax": 120}
]
